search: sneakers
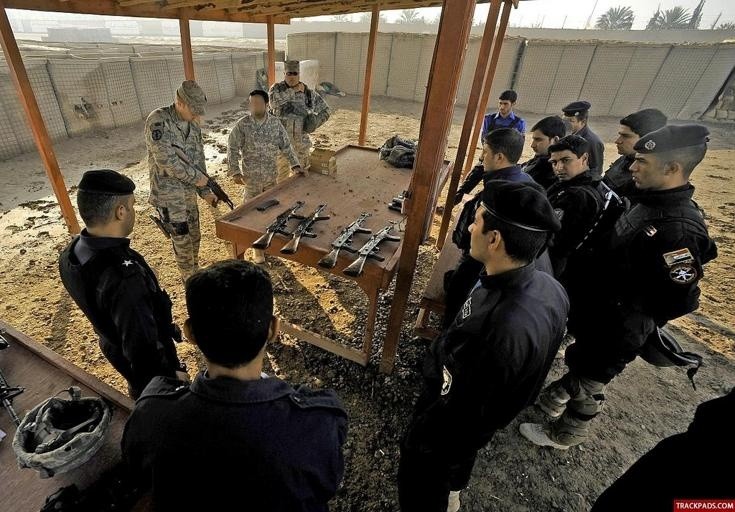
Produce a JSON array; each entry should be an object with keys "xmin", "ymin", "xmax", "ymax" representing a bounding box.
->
[
  {"xmin": 252, "ymin": 247, "xmax": 265, "ymax": 264},
  {"xmin": 534, "ymin": 388, "xmax": 567, "ymax": 417},
  {"xmin": 518, "ymin": 423, "xmax": 570, "ymax": 451}
]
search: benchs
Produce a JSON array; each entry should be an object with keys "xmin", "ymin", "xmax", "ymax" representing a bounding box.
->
[{"xmin": 411, "ymin": 193, "xmax": 477, "ymax": 341}]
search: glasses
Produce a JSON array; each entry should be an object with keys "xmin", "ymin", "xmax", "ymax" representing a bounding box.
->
[{"xmin": 285, "ymin": 72, "xmax": 299, "ymax": 77}]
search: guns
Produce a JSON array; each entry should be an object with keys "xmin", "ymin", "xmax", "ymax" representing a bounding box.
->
[
  {"xmin": 318, "ymin": 213, "xmax": 401, "ymax": 277},
  {"xmin": 252, "ymin": 200, "xmax": 330, "ymax": 252},
  {"xmin": 172, "ymin": 144, "xmax": 234, "ymax": 211},
  {"xmin": 0, "ymin": 333, "xmax": 25, "ymax": 427}
]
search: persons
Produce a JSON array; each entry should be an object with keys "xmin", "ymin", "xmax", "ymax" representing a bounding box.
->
[
  {"xmin": 226, "ymin": 90, "xmax": 309, "ymax": 204},
  {"xmin": 145, "ymin": 80, "xmax": 220, "ymax": 282},
  {"xmin": 59, "ymin": 170, "xmax": 191, "ymax": 399},
  {"xmin": 268, "ymin": 61, "xmax": 331, "ymax": 184},
  {"xmin": 120, "ymin": 259, "xmax": 348, "ymax": 512}
]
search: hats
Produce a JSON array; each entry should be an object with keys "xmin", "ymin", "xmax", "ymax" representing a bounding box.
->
[
  {"xmin": 77, "ymin": 169, "xmax": 135, "ymax": 196},
  {"xmin": 632, "ymin": 122, "xmax": 710, "ymax": 154},
  {"xmin": 480, "ymin": 179, "xmax": 562, "ymax": 233},
  {"xmin": 177, "ymin": 80, "xmax": 208, "ymax": 116},
  {"xmin": 284, "ymin": 60, "xmax": 300, "ymax": 73},
  {"xmin": 561, "ymin": 100, "xmax": 592, "ymax": 118}
]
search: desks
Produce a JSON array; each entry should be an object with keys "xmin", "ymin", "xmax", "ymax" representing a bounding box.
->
[
  {"xmin": 0, "ymin": 317, "xmax": 137, "ymax": 511},
  {"xmin": 214, "ymin": 144, "xmax": 456, "ymax": 365}
]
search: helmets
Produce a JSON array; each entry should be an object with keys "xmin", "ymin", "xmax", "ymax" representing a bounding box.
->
[
  {"xmin": 637, "ymin": 326, "xmax": 702, "ymax": 368},
  {"xmin": 11, "ymin": 385, "xmax": 113, "ymax": 478}
]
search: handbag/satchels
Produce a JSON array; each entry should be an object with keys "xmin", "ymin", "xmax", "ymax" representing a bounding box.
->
[{"xmin": 302, "ymin": 89, "xmax": 318, "ymax": 134}]
